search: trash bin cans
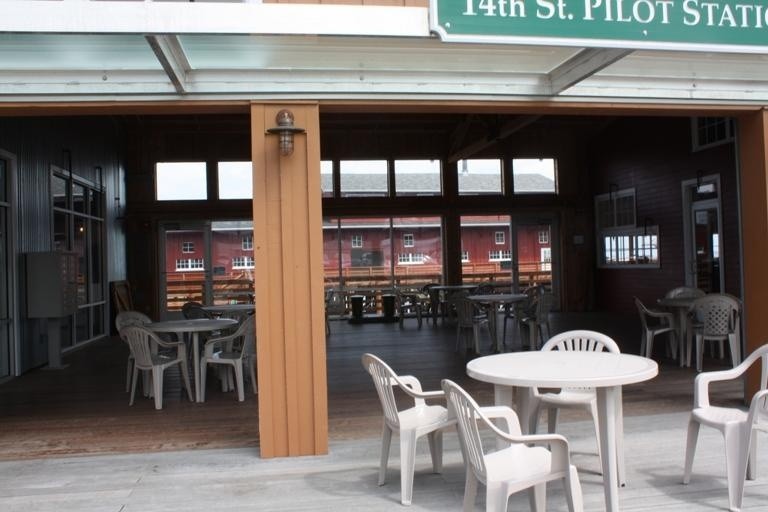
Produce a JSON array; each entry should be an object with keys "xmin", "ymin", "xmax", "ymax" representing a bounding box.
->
[
  {"xmin": 381, "ymin": 294, "xmax": 397, "ymax": 317},
  {"xmin": 350, "ymin": 295, "xmax": 364, "ymax": 316}
]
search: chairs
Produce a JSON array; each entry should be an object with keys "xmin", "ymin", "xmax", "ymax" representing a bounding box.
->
[
  {"xmin": 683, "ymin": 344, "xmax": 768, "ymax": 512},
  {"xmin": 361, "ymin": 352, "xmax": 467, "ymax": 506},
  {"xmin": 440, "ymin": 379, "xmax": 583, "ymax": 512},
  {"xmin": 396, "ymin": 284, "xmax": 555, "ymax": 355},
  {"xmin": 633, "ymin": 285, "xmax": 743, "ymax": 372},
  {"xmin": 529, "ymin": 329, "xmax": 625, "ymax": 488},
  {"xmin": 115, "ymin": 301, "xmax": 258, "ymax": 411}
]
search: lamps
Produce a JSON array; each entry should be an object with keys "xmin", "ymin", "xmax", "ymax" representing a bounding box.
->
[{"xmin": 266, "ymin": 109, "xmax": 306, "ymax": 157}]
